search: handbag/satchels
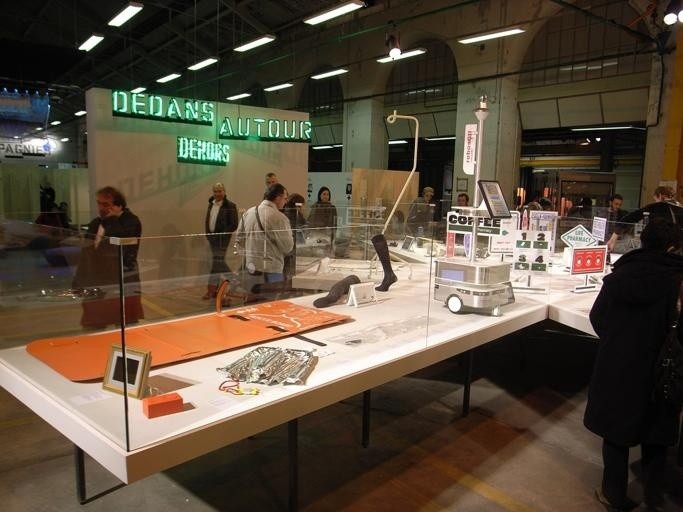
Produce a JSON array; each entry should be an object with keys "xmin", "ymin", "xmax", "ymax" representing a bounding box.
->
[
  {"xmin": 652, "ymin": 334, "xmax": 682, "ymax": 407},
  {"xmin": 283, "ymin": 253, "xmax": 295, "ymax": 274}
]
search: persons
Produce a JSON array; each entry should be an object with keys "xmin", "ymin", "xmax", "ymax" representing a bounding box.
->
[
  {"xmin": 570, "ymin": 197, "xmax": 592, "ymax": 219},
  {"xmin": 602, "ymin": 192, "xmax": 633, "ymax": 232},
  {"xmin": 405, "ymin": 187, "xmax": 436, "ymax": 240},
  {"xmin": 606, "ymin": 183, "xmax": 682, "ymax": 253},
  {"xmin": 367, "ymin": 233, "xmax": 399, "ymax": 290},
  {"xmin": 519, "ymin": 198, "xmax": 552, "ymax": 225},
  {"xmin": 71, "ymin": 185, "xmax": 144, "ymax": 331},
  {"xmin": 457, "ymin": 193, "xmax": 470, "ymax": 207},
  {"xmin": 581, "ymin": 219, "xmax": 681, "ymax": 506},
  {"xmin": 27, "ymin": 186, "xmax": 70, "ymax": 268},
  {"xmin": 199, "ymin": 172, "xmax": 339, "ymax": 302}
]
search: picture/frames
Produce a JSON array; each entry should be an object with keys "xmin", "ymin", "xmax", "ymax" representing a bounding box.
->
[
  {"xmin": 478, "ymin": 179, "xmax": 512, "ymax": 219},
  {"xmin": 103, "ymin": 346, "xmax": 150, "ymax": 399}
]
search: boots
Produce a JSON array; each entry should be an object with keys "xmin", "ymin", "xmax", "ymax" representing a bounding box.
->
[{"xmin": 372, "ymin": 234, "xmax": 397, "ymax": 291}]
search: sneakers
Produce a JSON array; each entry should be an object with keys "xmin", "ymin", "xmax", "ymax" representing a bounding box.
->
[
  {"xmin": 202, "ymin": 289, "xmax": 217, "ymax": 300},
  {"xmin": 642, "ymin": 488, "xmax": 665, "ymax": 511},
  {"xmin": 596, "ymin": 486, "xmax": 614, "ymax": 506}
]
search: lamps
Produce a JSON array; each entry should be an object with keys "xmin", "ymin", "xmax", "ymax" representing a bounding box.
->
[
  {"xmin": 662, "ymin": 0, "xmax": 683, "ymax": 25},
  {"xmin": 666, "ymin": 1, "xmax": 683, "ymax": 25}
]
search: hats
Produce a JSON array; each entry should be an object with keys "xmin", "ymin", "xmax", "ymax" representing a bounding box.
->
[
  {"xmin": 539, "ymin": 198, "xmax": 551, "ymax": 206},
  {"xmin": 422, "ymin": 187, "xmax": 434, "ymax": 197}
]
